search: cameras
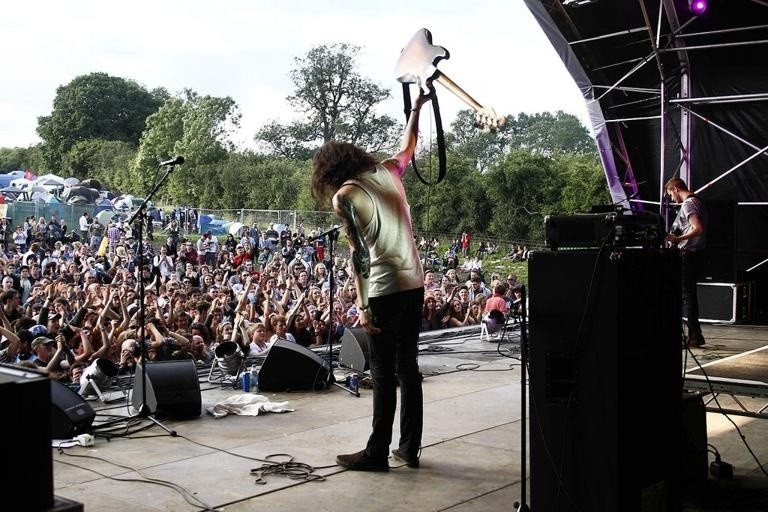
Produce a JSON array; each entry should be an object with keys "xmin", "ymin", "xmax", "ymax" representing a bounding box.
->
[
  {"xmin": 129, "ymin": 346, "xmax": 141, "ymax": 357},
  {"xmin": 314, "ymin": 310, "xmax": 323, "ymax": 321}
]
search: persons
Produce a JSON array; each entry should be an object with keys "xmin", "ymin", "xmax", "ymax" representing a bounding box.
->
[
  {"xmin": 309, "ymin": 78, "xmax": 436, "ymax": 472},
  {"xmin": 661, "ymin": 176, "xmax": 708, "ymax": 348}
]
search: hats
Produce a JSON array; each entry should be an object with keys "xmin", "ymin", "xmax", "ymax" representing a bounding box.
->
[
  {"xmin": 47, "ymin": 313, "xmax": 62, "ymax": 321},
  {"xmin": 31, "ymin": 336, "xmax": 53, "ymax": 350}
]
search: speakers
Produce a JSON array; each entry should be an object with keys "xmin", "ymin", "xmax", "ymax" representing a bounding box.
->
[
  {"xmin": 528, "ymin": 249, "xmax": 683, "ymax": 512},
  {"xmin": 131, "ymin": 359, "xmax": 202, "ymax": 415},
  {"xmin": 339, "ymin": 327, "xmax": 370, "ymax": 372},
  {"xmin": 51, "ymin": 379, "xmax": 97, "ymax": 439},
  {"xmin": 0, "ymin": 362, "xmax": 54, "ymax": 511},
  {"xmin": 257, "ymin": 338, "xmax": 334, "ymax": 392}
]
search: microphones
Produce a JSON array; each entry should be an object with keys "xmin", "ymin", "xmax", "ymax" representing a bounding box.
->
[{"xmin": 160, "ymin": 156, "xmax": 184, "ymax": 165}]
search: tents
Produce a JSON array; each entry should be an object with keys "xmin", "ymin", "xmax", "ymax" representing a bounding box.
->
[{"xmin": 61, "ymin": 176, "xmax": 147, "ymax": 215}]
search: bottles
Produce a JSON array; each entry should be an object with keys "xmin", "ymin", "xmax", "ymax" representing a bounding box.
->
[{"xmin": 251, "ymin": 364, "xmax": 258, "ymax": 386}]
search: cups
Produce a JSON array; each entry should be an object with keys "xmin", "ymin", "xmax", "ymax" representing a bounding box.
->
[
  {"xmin": 350, "ymin": 378, "xmax": 357, "ymax": 392},
  {"xmin": 242, "ymin": 373, "xmax": 250, "ymax": 392}
]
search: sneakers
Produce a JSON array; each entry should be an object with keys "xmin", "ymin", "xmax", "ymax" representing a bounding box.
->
[
  {"xmin": 683, "ymin": 332, "xmax": 708, "ymax": 351},
  {"xmin": 334, "ymin": 447, "xmax": 392, "ymax": 473},
  {"xmin": 392, "ymin": 446, "xmax": 420, "ymax": 470}
]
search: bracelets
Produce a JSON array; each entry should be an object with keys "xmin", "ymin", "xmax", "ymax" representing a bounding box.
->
[
  {"xmin": 675, "ymin": 236, "xmax": 678, "ymax": 242},
  {"xmin": 409, "ymin": 109, "xmax": 420, "ymax": 111}
]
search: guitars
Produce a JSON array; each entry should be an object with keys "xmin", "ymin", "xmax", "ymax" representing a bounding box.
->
[{"xmin": 395, "ymin": 27, "xmax": 483, "ymax": 115}]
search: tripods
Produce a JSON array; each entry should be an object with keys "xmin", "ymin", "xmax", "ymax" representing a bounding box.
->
[
  {"xmin": 91, "ymin": 166, "xmax": 179, "ymax": 437},
  {"xmin": 286, "ymin": 225, "xmax": 361, "ymax": 397}
]
